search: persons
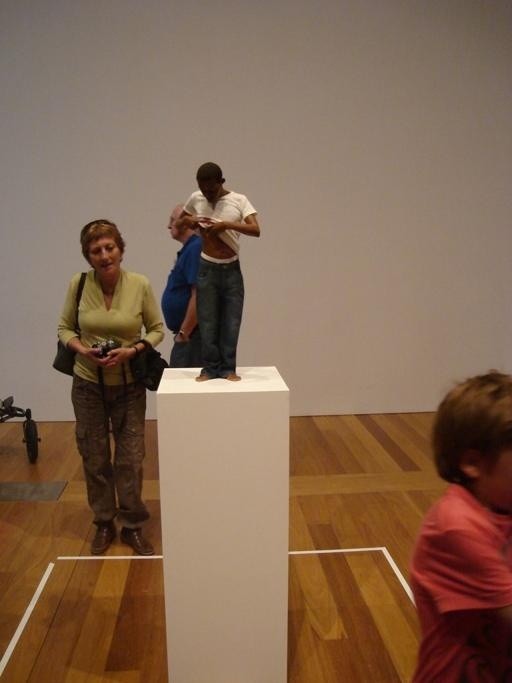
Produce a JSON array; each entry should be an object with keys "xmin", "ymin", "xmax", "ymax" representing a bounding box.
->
[
  {"xmin": 409, "ymin": 372, "xmax": 512, "ymax": 683},
  {"xmin": 53, "ymin": 220, "xmax": 165, "ymax": 556},
  {"xmin": 162, "ymin": 203, "xmax": 202, "ymax": 368},
  {"xmin": 176, "ymin": 162, "xmax": 261, "ymax": 381}
]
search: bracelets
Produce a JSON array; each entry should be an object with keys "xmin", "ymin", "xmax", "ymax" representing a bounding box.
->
[
  {"xmin": 134, "ymin": 346, "xmax": 139, "ymax": 358},
  {"xmin": 178, "ymin": 328, "xmax": 188, "ymax": 337}
]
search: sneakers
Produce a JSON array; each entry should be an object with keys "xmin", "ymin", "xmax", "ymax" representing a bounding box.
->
[
  {"xmin": 91, "ymin": 526, "xmax": 154, "ymax": 555},
  {"xmin": 196, "ymin": 372, "xmax": 241, "ymax": 382}
]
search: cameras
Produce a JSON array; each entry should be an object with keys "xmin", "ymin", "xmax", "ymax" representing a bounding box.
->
[{"xmin": 92, "ymin": 339, "xmax": 121, "ymax": 358}]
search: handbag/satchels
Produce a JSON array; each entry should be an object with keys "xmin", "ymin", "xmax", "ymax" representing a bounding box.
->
[
  {"xmin": 53, "ymin": 341, "xmax": 75, "ymax": 376},
  {"xmin": 129, "ymin": 340, "xmax": 169, "ymax": 391}
]
